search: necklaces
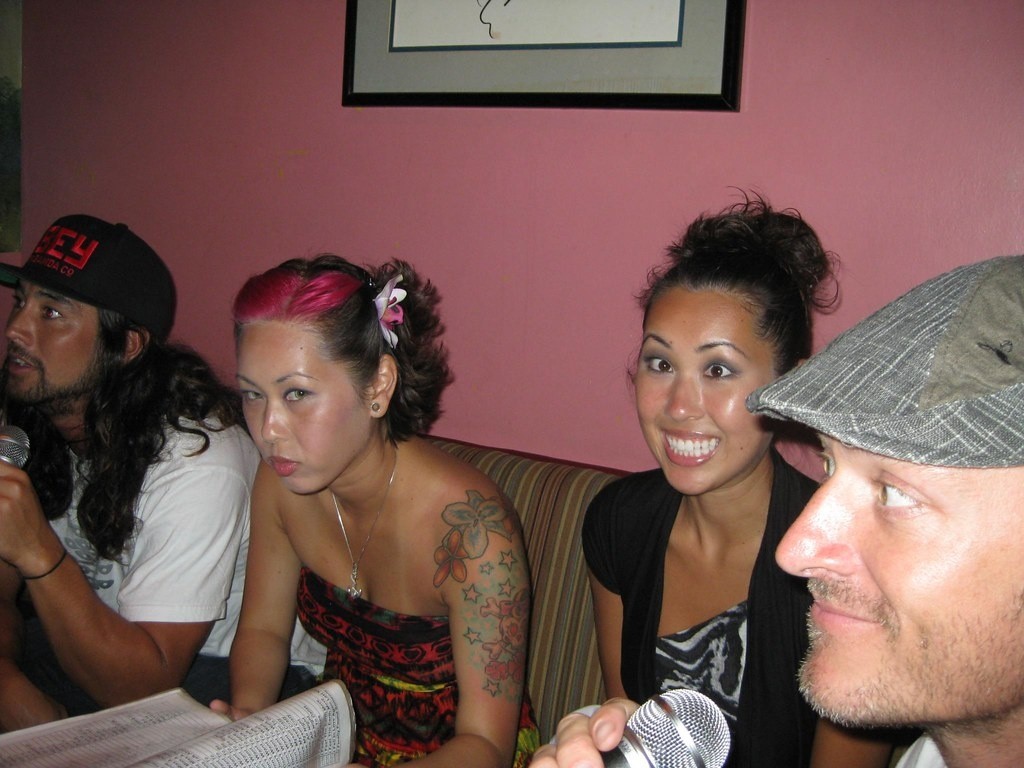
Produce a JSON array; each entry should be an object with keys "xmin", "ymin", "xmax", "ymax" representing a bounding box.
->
[{"xmin": 334, "ymin": 455, "xmax": 403, "ymax": 600}]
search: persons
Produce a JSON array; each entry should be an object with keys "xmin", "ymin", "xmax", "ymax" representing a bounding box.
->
[
  {"xmin": 580, "ymin": 202, "xmax": 900, "ymax": 767},
  {"xmin": 0, "ymin": 210, "xmax": 333, "ymax": 746},
  {"xmin": 524, "ymin": 252, "xmax": 1022, "ymax": 765},
  {"xmin": 210, "ymin": 254, "xmax": 542, "ymax": 768}
]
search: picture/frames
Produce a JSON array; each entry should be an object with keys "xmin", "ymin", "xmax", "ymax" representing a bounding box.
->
[{"xmin": 342, "ymin": 0, "xmax": 746, "ymax": 112}]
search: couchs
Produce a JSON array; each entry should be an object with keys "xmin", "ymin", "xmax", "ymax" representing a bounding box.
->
[{"xmin": 421, "ymin": 435, "xmax": 633, "ymax": 747}]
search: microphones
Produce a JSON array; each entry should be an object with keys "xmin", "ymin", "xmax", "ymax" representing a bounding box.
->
[
  {"xmin": 602, "ymin": 688, "xmax": 731, "ymax": 768},
  {"xmin": 0, "ymin": 425, "xmax": 30, "ymax": 469}
]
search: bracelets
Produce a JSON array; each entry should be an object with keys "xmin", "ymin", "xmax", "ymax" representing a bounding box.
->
[{"xmin": 23, "ymin": 547, "xmax": 67, "ymax": 580}]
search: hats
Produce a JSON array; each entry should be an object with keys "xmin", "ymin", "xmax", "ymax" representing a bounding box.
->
[
  {"xmin": 747, "ymin": 252, "xmax": 1024, "ymax": 470},
  {"xmin": 0, "ymin": 214, "xmax": 177, "ymax": 340}
]
